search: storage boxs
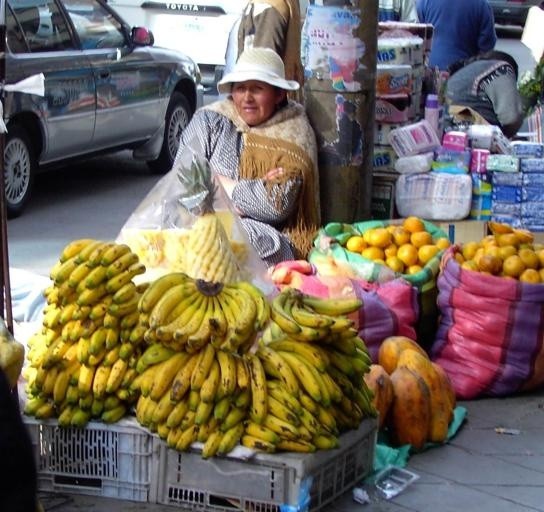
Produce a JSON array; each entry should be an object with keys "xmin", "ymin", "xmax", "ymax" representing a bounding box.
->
[
  {"xmin": 370, "ymin": 173, "xmax": 397, "ymax": 222},
  {"xmin": 429, "ymin": 216, "xmax": 489, "ymax": 248}
]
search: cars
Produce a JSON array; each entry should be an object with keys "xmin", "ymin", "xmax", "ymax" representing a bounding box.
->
[{"xmin": 486, "ymin": 0, "xmax": 544, "ymax": 29}]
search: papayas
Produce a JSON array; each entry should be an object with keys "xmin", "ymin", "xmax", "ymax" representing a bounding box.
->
[{"xmin": 363, "ymin": 336, "xmax": 456, "ymax": 452}]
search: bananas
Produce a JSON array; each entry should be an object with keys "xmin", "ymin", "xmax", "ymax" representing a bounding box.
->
[{"xmin": 22, "ymin": 238, "xmax": 379, "ymax": 462}]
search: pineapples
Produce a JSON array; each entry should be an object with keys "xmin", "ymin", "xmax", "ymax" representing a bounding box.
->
[{"xmin": 134, "ymin": 208, "xmax": 248, "ymax": 284}]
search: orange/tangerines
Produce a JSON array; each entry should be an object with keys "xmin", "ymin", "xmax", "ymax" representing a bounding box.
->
[{"xmin": 346, "ymin": 216, "xmax": 544, "ymax": 286}]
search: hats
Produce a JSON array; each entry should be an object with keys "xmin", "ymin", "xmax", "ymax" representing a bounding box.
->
[{"xmin": 215, "ymin": 45, "xmax": 302, "ymax": 99}]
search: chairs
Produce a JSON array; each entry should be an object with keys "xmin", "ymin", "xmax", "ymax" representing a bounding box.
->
[{"xmin": 11, "ymin": 5, "xmax": 59, "ymax": 50}]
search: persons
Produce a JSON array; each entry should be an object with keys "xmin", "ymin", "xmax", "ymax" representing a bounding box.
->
[
  {"xmin": 233, "ymin": 1, "xmax": 305, "ymax": 105},
  {"xmin": 413, "ymin": 0, "xmax": 499, "ymax": 76},
  {"xmin": 435, "ymin": 50, "xmax": 524, "ymax": 143},
  {"xmin": 162, "ymin": 48, "xmax": 321, "ymax": 269}
]
